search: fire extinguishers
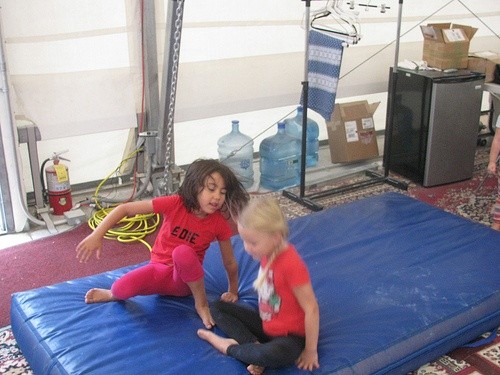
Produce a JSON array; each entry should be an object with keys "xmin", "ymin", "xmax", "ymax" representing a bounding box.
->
[{"xmin": 40, "ymin": 150, "xmax": 73, "ymax": 215}]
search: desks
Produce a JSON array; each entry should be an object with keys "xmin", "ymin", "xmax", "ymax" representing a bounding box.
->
[
  {"xmin": 479, "ymin": 82, "xmax": 500, "ymax": 138},
  {"xmin": 382, "ymin": 66, "xmax": 486, "ymax": 187}
]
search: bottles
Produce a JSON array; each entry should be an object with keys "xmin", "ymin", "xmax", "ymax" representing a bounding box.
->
[
  {"xmin": 259, "ymin": 122, "xmax": 301, "ymax": 191},
  {"xmin": 217, "ymin": 120, "xmax": 254, "ymax": 188},
  {"xmin": 284, "ymin": 106, "xmax": 319, "ymax": 168}
]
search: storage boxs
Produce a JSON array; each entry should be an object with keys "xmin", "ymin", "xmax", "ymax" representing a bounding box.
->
[
  {"xmin": 325, "ymin": 101, "xmax": 383, "ymax": 164},
  {"xmin": 420, "ymin": 22, "xmax": 479, "ymax": 70},
  {"xmin": 467, "ymin": 49, "xmax": 500, "ymax": 82}
]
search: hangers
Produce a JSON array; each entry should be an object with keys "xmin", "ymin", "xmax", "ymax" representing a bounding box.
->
[{"xmin": 301, "ymin": 0, "xmax": 390, "ymax": 47}]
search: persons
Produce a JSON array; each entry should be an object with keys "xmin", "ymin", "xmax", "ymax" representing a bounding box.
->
[
  {"xmin": 197, "ymin": 198, "xmax": 320, "ymax": 375},
  {"xmin": 76, "ymin": 159, "xmax": 248, "ymax": 330},
  {"xmin": 487, "ymin": 115, "xmax": 500, "ymax": 230}
]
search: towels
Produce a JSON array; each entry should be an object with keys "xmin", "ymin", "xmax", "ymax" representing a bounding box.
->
[{"xmin": 298, "ymin": 30, "xmax": 346, "ymax": 121}]
